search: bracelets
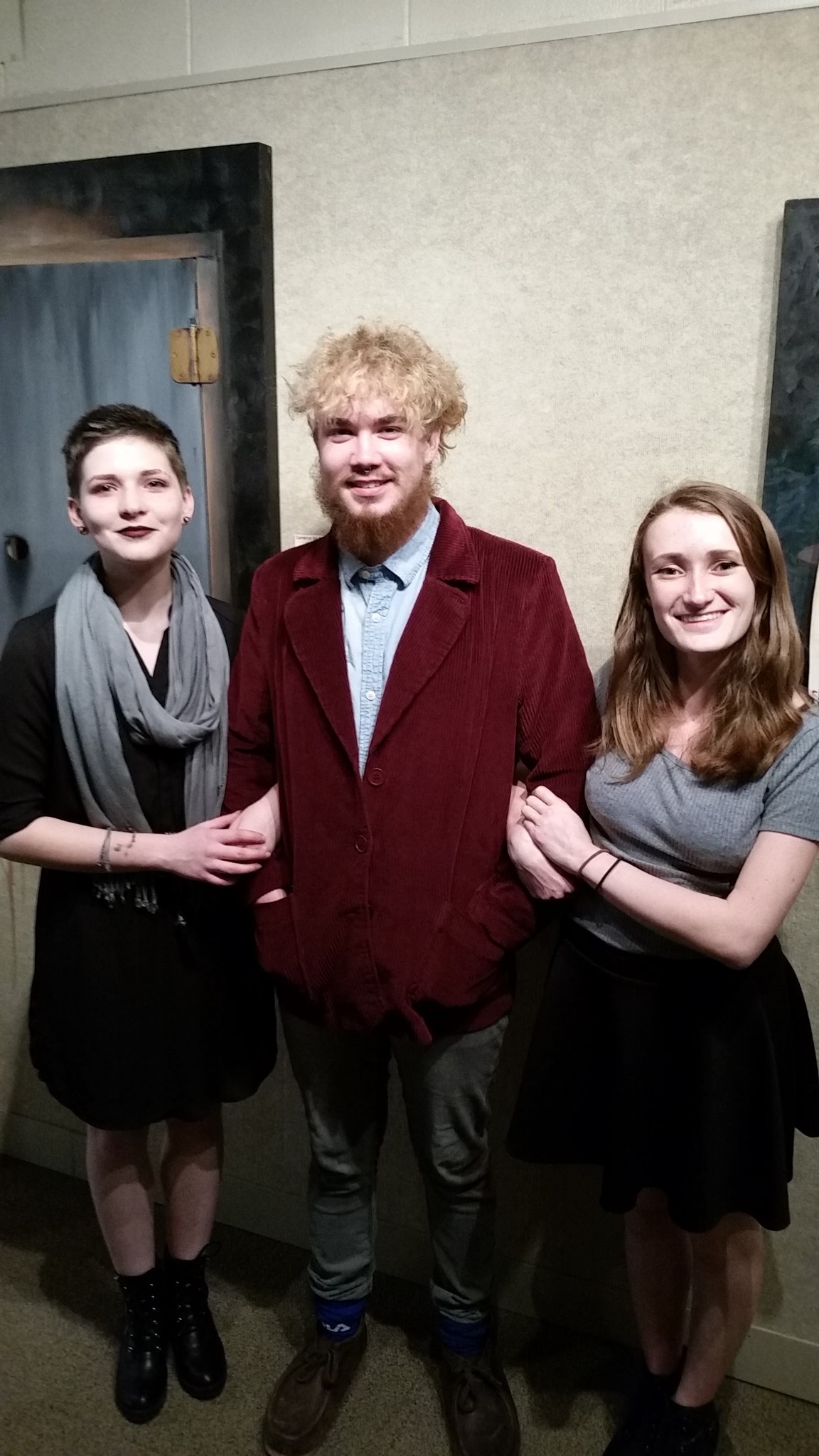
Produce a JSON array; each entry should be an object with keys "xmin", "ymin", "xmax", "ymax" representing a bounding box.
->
[
  {"xmin": 593, "ymin": 857, "xmax": 624, "ymax": 891},
  {"xmin": 98, "ymin": 827, "xmax": 114, "ymax": 875},
  {"xmin": 576, "ymin": 847, "xmax": 610, "ymax": 876}
]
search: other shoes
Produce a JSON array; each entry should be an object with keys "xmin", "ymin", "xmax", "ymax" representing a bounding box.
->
[
  {"xmin": 671, "ymin": 1402, "xmax": 721, "ymax": 1456},
  {"xmin": 603, "ymin": 1369, "xmax": 678, "ymax": 1455}
]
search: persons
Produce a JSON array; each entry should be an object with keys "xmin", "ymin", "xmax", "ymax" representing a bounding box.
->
[
  {"xmin": 504, "ymin": 481, "xmax": 819, "ymax": 1455},
  {"xmin": 232, "ymin": 329, "xmax": 600, "ymax": 1456},
  {"xmin": 0, "ymin": 402, "xmax": 278, "ymax": 1415}
]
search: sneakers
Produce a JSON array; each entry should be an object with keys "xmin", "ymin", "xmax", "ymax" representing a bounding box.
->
[
  {"xmin": 265, "ymin": 1316, "xmax": 369, "ymax": 1456},
  {"xmin": 446, "ymin": 1345, "xmax": 520, "ymax": 1456}
]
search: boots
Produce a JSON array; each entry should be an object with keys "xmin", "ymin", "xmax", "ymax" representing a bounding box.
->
[
  {"xmin": 115, "ymin": 1259, "xmax": 168, "ymax": 1423},
  {"xmin": 168, "ymin": 1244, "xmax": 227, "ymax": 1401}
]
